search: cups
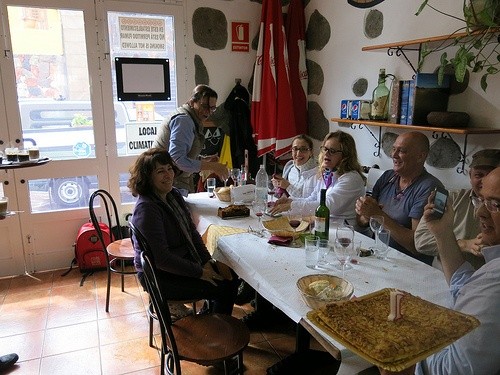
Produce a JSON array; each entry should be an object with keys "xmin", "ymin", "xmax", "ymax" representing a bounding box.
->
[
  {"xmin": 29, "ymin": 146, "xmax": 40, "ymax": 161},
  {"xmin": 206, "ymin": 178, "xmax": 215, "ymax": 198},
  {"xmin": 5, "ymin": 148, "xmax": 18, "ymax": 162},
  {"xmin": 349, "ymin": 240, "xmax": 362, "ymax": 264},
  {"xmin": 0, "ymin": 196, "xmax": 8, "ymax": 216},
  {"xmin": 18, "ymin": 149, "xmax": 30, "ymax": 163},
  {"xmin": 375, "ymin": 229, "xmax": 390, "ymax": 260},
  {"xmin": 317, "ymin": 240, "xmax": 331, "ymax": 265},
  {"xmin": 305, "ymin": 235, "xmax": 319, "ymax": 268}
]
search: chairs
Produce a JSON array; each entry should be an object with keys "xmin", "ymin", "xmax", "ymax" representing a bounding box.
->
[{"xmin": 89, "ymin": 189, "xmax": 252, "ymax": 375}]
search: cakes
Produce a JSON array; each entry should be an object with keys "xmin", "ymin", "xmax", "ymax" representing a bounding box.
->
[{"xmin": 313, "ymin": 288, "xmax": 472, "ymax": 364}]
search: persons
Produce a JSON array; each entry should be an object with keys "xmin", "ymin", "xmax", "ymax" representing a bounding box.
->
[
  {"xmin": 268, "ymin": 130, "xmax": 367, "ymax": 229},
  {"xmin": 378, "ymin": 164, "xmax": 499, "ymax": 374},
  {"xmin": 412, "ymin": 148, "xmax": 500, "ymax": 273},
  {"xmin": 125, "ymin": 147, "xmax": 239, "ymax": 315},
  {"xmin": 152, "ymin": 84, "xmax": 229, "ymax": 191},
  {"xmin": 270, "ymin": 134, "xmax": 320, "ymax": 198},
  {"xmin": 354, "ymin": 131, "xmax": 450, "ymax": 263}
]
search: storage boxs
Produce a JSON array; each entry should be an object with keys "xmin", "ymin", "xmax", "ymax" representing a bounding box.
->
[{"xmin": 341, "ymin": 99, "xmax": 371, "ymax": 122}]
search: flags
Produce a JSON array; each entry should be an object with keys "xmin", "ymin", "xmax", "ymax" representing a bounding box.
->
[{"xmin": 250, "ymin": 0, "xmax": 309, "ymax": 172}]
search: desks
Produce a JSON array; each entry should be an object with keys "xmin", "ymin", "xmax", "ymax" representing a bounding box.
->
[
  {"xmin": 0, "ymin": 158, "xmax": 50, "ymax": 282},
  {"xmin": 183, "ymin": 184, "xmax": 456, "ymax": 375}
]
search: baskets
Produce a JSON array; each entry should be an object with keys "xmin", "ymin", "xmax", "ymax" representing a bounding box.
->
[
  {"xmin": 296, "ymin": 273, "xmax": 354, "ymax": 310},
  {"xmin": 262, "ymin": 216, "xmax": 310, "ymax": 238}
]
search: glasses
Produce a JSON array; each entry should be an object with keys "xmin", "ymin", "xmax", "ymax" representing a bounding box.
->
[
  {"xmin": 290, "ymin": 147, "xmax": 311, "ymax": 153},
  {"xmin": 470, "ymin": 195, "xmax": 500, "ymax": 214},
  {"xmin": 319, "ymin": 145, "xmax": 342, "ymax": 156}
]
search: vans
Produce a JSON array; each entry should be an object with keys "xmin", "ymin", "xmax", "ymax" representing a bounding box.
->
[{"xmin": 19, "ymin": 99, "xmax": 167, "ymax": 208}]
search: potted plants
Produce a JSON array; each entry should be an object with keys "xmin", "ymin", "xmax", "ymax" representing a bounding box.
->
[{"xmin": 415, "ymin": 0, "xmax": 500, "ymax": 92}]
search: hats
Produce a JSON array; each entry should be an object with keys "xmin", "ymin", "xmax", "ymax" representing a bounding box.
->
[{"xmin": 469, "ymin": 148, "xmax": 500, "ymax": 167}]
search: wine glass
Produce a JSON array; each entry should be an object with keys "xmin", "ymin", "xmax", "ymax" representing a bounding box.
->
[
  {"xmin": 334, "ymin": 239, "xmax": 353, "ymax": 278},
  {"xmin": 336, "ymin": 225, "xmax": 354, "ymax": 255},
  {"xmin": 252, "ymin": 200, "xmax": 266, "ymax": 234},
  {"xmin": 370, "ymin": 215, "xmax": 384, "ymax": 249},
  {"xmin": 230, "ymin": 169, "xmax": 240, "ymax": 187},
  {"xmin": 287, "ymin": 208, "xmax": 302, "ymax": 248},
  {"xmin": 221, "ymin": 171, "xmax": 230, "ymax": 187},
  {"xmin": 267, "ymin": 181, "xmax": 277, "ymax": 207}
]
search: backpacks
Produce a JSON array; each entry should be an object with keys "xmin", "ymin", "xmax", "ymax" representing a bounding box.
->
[{"xmin": 72, "ymin": 221, "xmax": 118, "ymax": 272}]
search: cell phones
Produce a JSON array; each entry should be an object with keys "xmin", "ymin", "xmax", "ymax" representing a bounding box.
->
[{"xmin": 431, "ymin": 185, "xmax": 449, "ymax": 218}]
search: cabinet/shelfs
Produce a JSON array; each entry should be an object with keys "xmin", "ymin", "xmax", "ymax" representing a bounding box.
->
[{"xmin": 328, "ymin": 28, "xmax": 500, "ymax": 177}]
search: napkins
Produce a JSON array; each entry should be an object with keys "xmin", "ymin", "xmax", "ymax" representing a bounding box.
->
[
  {"xmin": 268, "ymin": 235, "xmax": 293, "ymax": 247},
  {"xmin": 274, "ymin": 173, "xmax": 290, "ymax": 197},
  {"xmin": 201, "ymin": 223, "xmax": 247, "ymax": 256}
]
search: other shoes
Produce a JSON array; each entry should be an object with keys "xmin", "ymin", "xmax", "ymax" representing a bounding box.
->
[
  {"xmin": 237, "ymin": 311, "xmax": 274, "ymax": 331},
  {"xmin": 234, "ymin": 284, "xmax": 253, "ymax": 306},
  {"xmin": 0, "ymin": 353, "xmax": 19, "ymax": 372}
]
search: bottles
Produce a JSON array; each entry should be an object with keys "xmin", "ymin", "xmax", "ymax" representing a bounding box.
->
[
  {"xmin": 241, "ymin": 164, "xmax": 246, "ymax": 185},
  {"xmin": 370, "ymin": 68, "xmax": 390, "ymax": 121},
  {"xmin": 314, "ymin": 189, "xmax": 330, "ymax": 247},
  {"xmin": 255, "ymin": 165, "xmax": 269, "ymax": 200}
]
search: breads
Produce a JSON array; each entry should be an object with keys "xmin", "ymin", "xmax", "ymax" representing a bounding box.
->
[
  {"xmin": 218, "ymin": 187, "xmax": 230, "ymax": 192},
  {"xmin": 218, "ymin": 204, "xmax": 250, "ymax": 219}
]
